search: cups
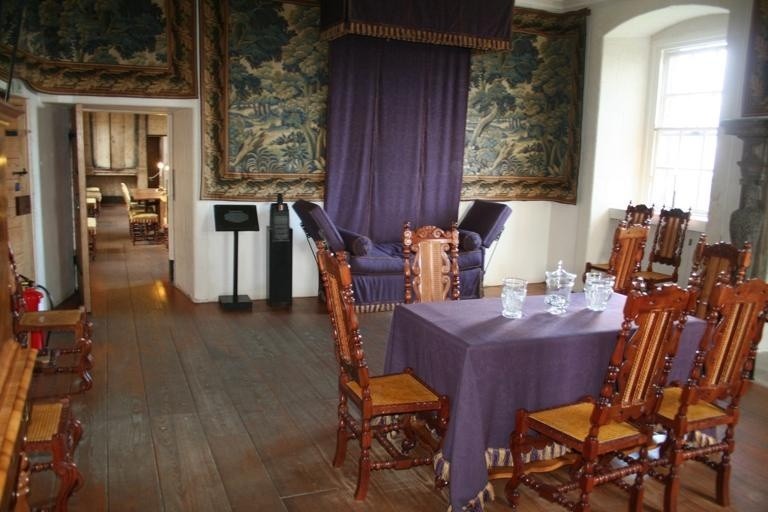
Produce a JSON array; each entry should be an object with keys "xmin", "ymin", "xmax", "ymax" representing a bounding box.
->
[
  {"xmin": 501, "ymin": 277, "xmax": 526, "ymax": 320},
  {"xmin": 583, "ymin": 273, "xmax": 615, "ymax": 313}
]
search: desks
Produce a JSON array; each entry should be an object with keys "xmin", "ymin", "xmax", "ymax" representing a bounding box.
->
[
  {"xmin": 129, "ymin": 188, "xmax": 162, "ymax": 213},
  {"xmin": 85, "ymin": 187, "xmax": 103, "ymax": 262}
]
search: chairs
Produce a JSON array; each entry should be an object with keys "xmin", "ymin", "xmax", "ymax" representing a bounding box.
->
[{"xmin": 120, "ymin": 182, "xmax": 160, "ymax": 246}]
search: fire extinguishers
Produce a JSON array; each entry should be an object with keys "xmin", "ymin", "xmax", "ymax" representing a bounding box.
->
[{"xmin": 11, "ymin": 274, "xmax": 55, "ymax": 351}]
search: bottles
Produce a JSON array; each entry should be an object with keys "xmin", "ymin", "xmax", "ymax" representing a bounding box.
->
[{"xmin": 542, "ymin": 260, "xmax": 576, "ymax": 316}]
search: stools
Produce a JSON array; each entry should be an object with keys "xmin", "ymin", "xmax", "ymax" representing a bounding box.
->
[
  {"xmin": 25, "ymin": 393, "xmax": 83, "ymax": 512},
  {"xmin": 17, "ymin": 307, "xmax": 95, "ymax": 393}
]
